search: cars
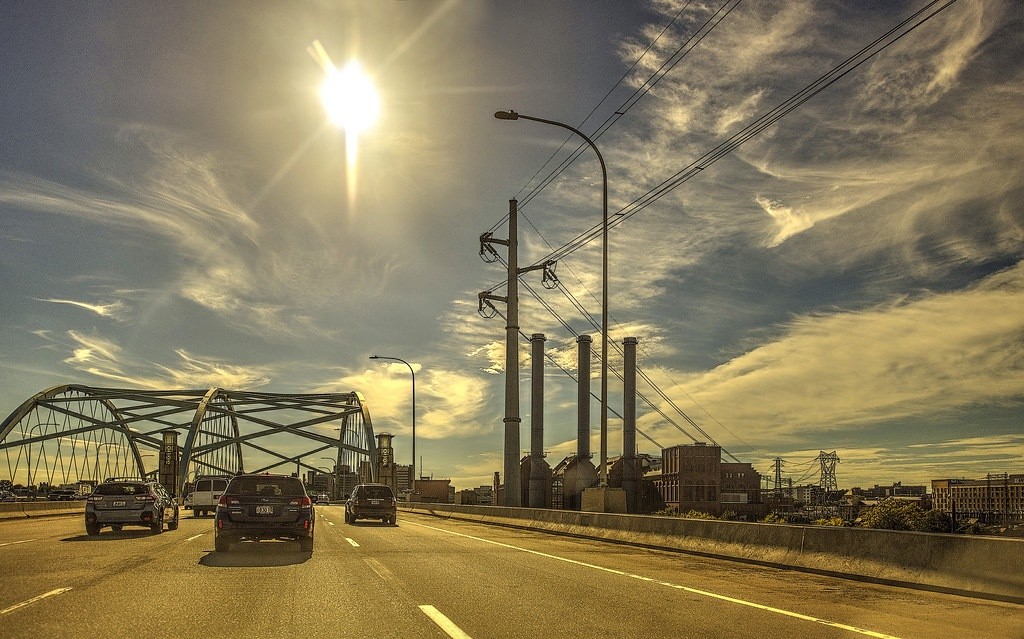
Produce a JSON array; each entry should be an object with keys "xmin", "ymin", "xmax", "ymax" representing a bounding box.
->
[
  {"xmin": 46, "ymin": 490, "xmax": 91, "ymax": 501},
  {"xmin": 316, "ymin": 495, "xmax": 330, "ymax": 505},
  {"xmin": 184, "ymin": 492, "xmax": 193, "ymax": 510},
  {"xmin": 344, "ymin": 483, "xmax": 398, "ymax": 525}
]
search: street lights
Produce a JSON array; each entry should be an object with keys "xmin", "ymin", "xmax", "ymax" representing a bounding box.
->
[
  {"xmin": 137, "ymin": 454, "xmax": 155, "ymax": 482},
  {"xmin": 368, "ymin": 355, "xmax": 417, "ymax": 480},
  {"xmin": 334, "ymin": 428, "xmax": 360, "ymax": 483},
  {"xmin": 493, "ymin": 109, "xmax": 611, "ymax": 487},
  {"xmin": 318, "ymin": 467, "xmax": 331, "ymax": 475},
  {"xmin": 27, "ymin": 423, "xmax": 61, "ymax": 493},
  {"xmin": 95, "ymin": 442, "xmax": 120, "ymax": 485},
  {"xmin": 321, "ymin": 456, "xmax": 336, "ymax": 466}
]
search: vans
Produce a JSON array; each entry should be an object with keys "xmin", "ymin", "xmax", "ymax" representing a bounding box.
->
[{"xmin": 193, "ymin": 476, "xmax": 232, "ymax": 517}]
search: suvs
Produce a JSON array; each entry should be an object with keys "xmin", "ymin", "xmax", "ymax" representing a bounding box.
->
[
  {"xmin": 85, "ymin": 476, "xmax": 180, "ymax": 535},
  {"xmin": 214, "ymin": 471, "xmax": 317, "ymax": 553}
]
